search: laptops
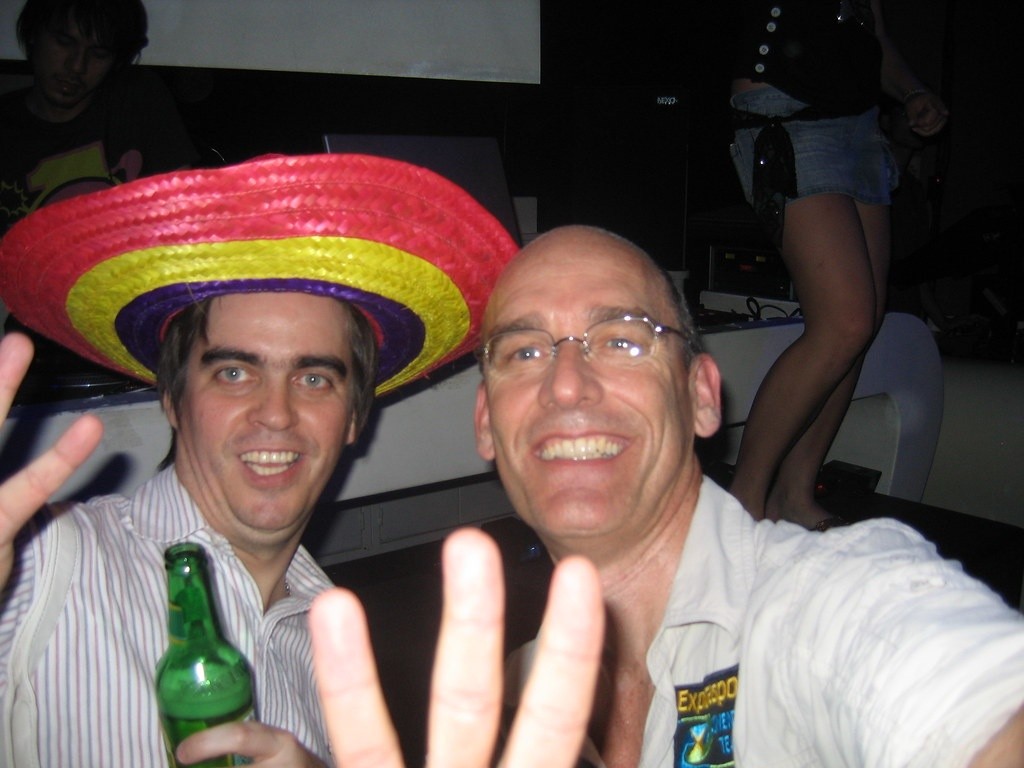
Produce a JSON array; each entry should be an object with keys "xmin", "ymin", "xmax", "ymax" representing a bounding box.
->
[{"xmin": 323, "ymin": 132, "xmax": 521, "ymax": 247}]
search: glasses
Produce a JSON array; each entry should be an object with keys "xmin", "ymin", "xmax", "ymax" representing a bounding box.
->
[{"xmin": 478, "ymin": 316, "xmax": 701, "ymax": 381}]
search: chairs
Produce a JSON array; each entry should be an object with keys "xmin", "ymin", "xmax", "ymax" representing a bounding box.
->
[{"xmin": 323, "ymin": 133, "xmax": 519, "ymax": 243}]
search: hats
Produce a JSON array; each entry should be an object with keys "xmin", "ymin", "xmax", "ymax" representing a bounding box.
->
[{"xmin": 0, "ymin": 152, "xmax": 519, "ymax": 396}]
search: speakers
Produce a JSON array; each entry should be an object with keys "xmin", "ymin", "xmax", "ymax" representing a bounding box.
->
[{"xmin": 497, "ymin": 79, "xmax": 689, "ymax": 274}]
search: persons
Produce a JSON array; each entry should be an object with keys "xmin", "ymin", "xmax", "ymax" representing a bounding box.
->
[
  {"xmin": 728, "ymin": 0, "xmax": 948, "ymax": 528},
  {"xmin": 0, "ymin": 153, "xmax": 521, "ymax": 768},
  {"xmin": 309, "ymin": 224, "xmax": 1024, "ymax": 768},
  {"xmin": 1, "ymin": 0, "xmax": 200, "ymax": 228}
]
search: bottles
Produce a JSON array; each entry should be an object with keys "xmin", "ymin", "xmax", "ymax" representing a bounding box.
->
[{"xmin": 152, "ymin": 541, "xmax": 266, "ymax": 768}]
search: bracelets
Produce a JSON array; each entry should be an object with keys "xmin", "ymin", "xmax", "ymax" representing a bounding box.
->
[{"xmin": 903, "ymin": 89, "xmax": 927, "ymax": 103}]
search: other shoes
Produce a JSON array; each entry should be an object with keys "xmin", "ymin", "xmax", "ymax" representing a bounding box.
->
[{"xmin": 811, "ymin": 515, "xmax": 852, "ymax": 532}]
